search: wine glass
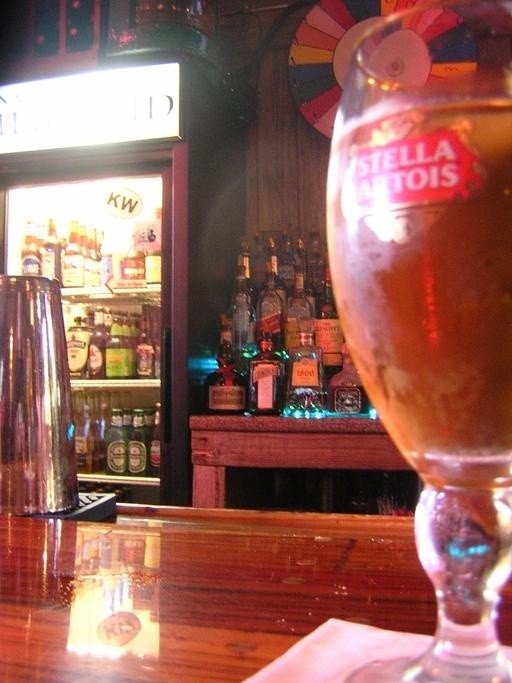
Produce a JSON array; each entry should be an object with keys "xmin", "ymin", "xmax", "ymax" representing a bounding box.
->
[{"xmin": 326, "ymin": 1, "xmax": 512, "ymax": 683}]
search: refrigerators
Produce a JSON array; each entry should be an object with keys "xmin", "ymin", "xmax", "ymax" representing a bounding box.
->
[{"xmin": 0, "ymin": 56, "xmax": 245, "ymax": 507}]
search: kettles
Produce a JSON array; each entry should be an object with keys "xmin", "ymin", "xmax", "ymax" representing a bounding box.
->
[{"xmin": 0, "ymin": 273, "xmax": 80, "ymax": 516}]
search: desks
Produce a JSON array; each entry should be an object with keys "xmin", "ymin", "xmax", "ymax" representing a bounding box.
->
[{"xmin": 182, "ymin": 407, "xmax": 426, "ymax": 514}]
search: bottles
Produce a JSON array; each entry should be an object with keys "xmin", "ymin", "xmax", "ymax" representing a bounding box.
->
[
  {"xmin": 14, "ymin": 209, "xmax": 107, "ymax": 286},
  {"xmin": 71, "ymin": 387, "xmax": 161, "ymax": 478},
  {"xmin": 60, "ymin": 296, "xmax": 162, "ymax": 380},
  {"xmin": 200, "ymin": 228, "xmax": 370, "ymax": 418}
]
